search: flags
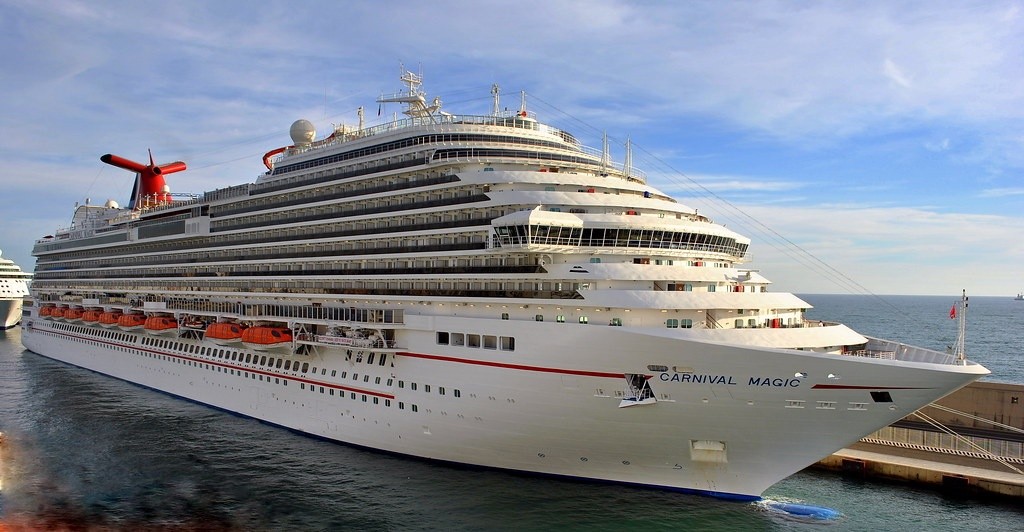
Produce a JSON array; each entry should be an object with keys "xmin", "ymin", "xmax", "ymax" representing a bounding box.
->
[{"xmin": 949, "ymin": 305, "xmax": 956, "ymax": 319}]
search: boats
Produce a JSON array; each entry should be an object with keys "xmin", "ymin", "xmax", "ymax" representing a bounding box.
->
[
  {"xmin": 117, "ymin": 310, "xmax": 148, "ymax": 332},
  {"xmin": 204, "ymin": 321, "xmax": 246, "ymax": 344},
  {"xmin": 0, "ymin": 249, "xmax": 36, "ymax": 332},
  {"xmin": 63, "ymin": 306, "xmax": 85, "ymax": 324},
  {"xmin": 241, "ymin": 324, "xmax": 294, "ymax": 351},
  {"xmin": 41, "ymin": 304, "xmax": 56, "ymax": 320},
  {"xmin": 81, "ymin": 309, "xmax": 105, "ymax": 327},
  {"xmin": 143, "ymin": 312, "xmax": 178, "ymax": 337},
  {"xmin": 97, "ymin": 310, "xmax": 123, "ymax": 329},
  {"xmin": 183, "ymin": 316, "xmax": 208, "ymax": 329},
  {"xmin": 50, "ymin": 305, "xmax": 69, "ymax": 322}
]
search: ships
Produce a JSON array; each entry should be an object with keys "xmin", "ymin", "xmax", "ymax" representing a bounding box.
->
[{"xmin": 19, "ymin": 59, "xmax": 994, "ymax": 506}]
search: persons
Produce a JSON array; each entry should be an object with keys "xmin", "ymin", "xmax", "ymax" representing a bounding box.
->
[
  {"xmin": 818, "ymin": 320, "xmax": 826, "ymax": 327},
  {"xmin": 200, "ymin": 318, "xmax": 217, "ymax": 327},
  {"xmin": 239, "ymin": 320, "xmax": 254, "ymax": 329}
]
